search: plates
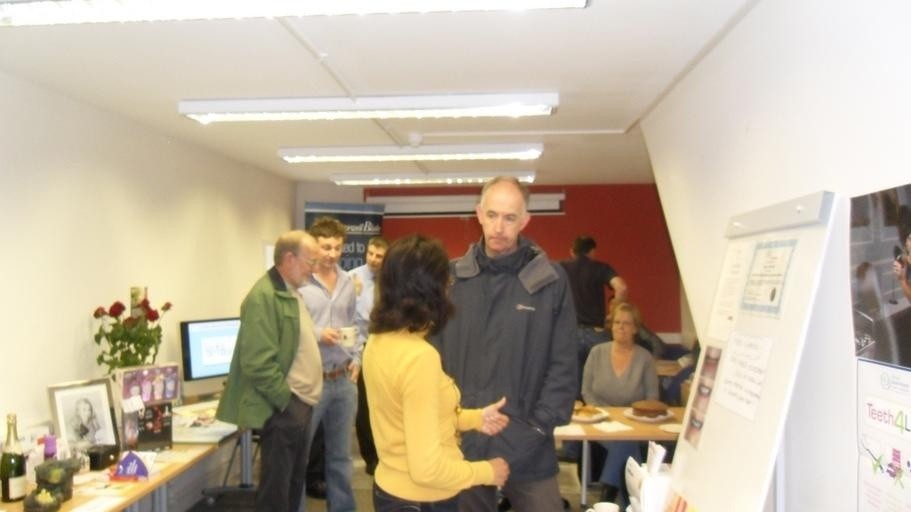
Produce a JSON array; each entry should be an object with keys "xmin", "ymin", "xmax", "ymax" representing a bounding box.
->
[
  {"xmin": 624, "ymin": 408, "xmax": 675, "ymax": 421},
  {"xmin": 571, "ymin": 407, "xmax": 609, "ymax": 421}
]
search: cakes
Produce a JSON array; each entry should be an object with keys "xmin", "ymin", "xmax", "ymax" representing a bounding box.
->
[{"xmin": 632, "ymin": 400, "xmax": 668, "ymax": 418}]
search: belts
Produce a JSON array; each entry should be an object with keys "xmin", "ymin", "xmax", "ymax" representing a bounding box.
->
[{"xmin": 322, "ymin": 369, "xmax": 346, "ymax": 381}]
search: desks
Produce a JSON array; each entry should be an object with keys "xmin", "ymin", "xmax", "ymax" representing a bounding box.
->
[
  {"xmin": 527, "ymin": 359, "xmax": 694, "ymax": 507},
  {"xmin": 2, "ymin": 400, "xmax": 256, "ymax": 512}
]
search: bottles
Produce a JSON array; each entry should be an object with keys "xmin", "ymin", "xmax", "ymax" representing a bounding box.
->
[{"xmin": 0, "ymin": 413, "xmax": 28, "ymax": 502}]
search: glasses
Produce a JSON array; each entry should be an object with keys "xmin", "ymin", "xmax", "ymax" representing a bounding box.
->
[{"xmin": 301, "ymin": 256, "xmax": 320, "ymax": 268}]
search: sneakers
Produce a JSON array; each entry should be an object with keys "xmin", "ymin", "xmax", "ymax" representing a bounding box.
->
[{"xmin": 306, "ymin": 478, "xmax": 328, "ymax": 499}]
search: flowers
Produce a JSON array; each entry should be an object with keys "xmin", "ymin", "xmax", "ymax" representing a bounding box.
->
[{"xmin": 88, "ymin": 283, "xmax": 172, "ymax": 375}]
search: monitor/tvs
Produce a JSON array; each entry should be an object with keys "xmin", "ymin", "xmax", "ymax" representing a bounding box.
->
[{"xmin": 180, "ymin": 317, "xmax": 241, "ymax": 381}]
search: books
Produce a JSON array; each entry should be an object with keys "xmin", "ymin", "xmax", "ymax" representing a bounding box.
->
[{"xmin": 172, "ymin": 416, "xmax": 239, "ymax": 444}]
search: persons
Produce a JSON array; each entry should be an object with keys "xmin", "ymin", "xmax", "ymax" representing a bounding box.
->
[
  {"xmin": 301, "ymin": 214, "xmax": 359, "ymax": 512},
  {"xmin": 683, "ymin": 346, "xmax": 722, "ymax": 449},
  {"xmin": 891, "ymin": 226, "xmax": 911, "ymax": 305},
  {"xmin": 421, "ymin": 174, "xmax": 577, "ymax": 512},
  {"xmin": 554, "ymin": 235, "xmax": 630, "ymax": 360},
  {"xmin": 347, "ymin": 238, "xmax": 387, "ymax": 475},
  {"xmin": 581, "ymin": 302, "xmax": 659, "ymax": 504},
  {"xmin": 601, "ymin": 294, "xmax": 669, "ymax": 361},
  {"xmin": 64, "ymin": 400, "xmax": 96, "ymax": 449},
  {"xmin": 361, "ymin": 230, "xmax": 510, "ymax": 512},
  {"xmin": 214, "ymin": 226, "xmax": 324, "ymax": 512}
]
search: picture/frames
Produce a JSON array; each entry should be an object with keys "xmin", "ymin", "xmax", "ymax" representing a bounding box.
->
[{"xmin": 45, "ymin": 379, "xmax": 121, "ymax": 454}]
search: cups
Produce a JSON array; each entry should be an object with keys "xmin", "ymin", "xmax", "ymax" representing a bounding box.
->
[{"xmin": 336, "ymin": 328, "xmax": 356, "ymax": 347}]
